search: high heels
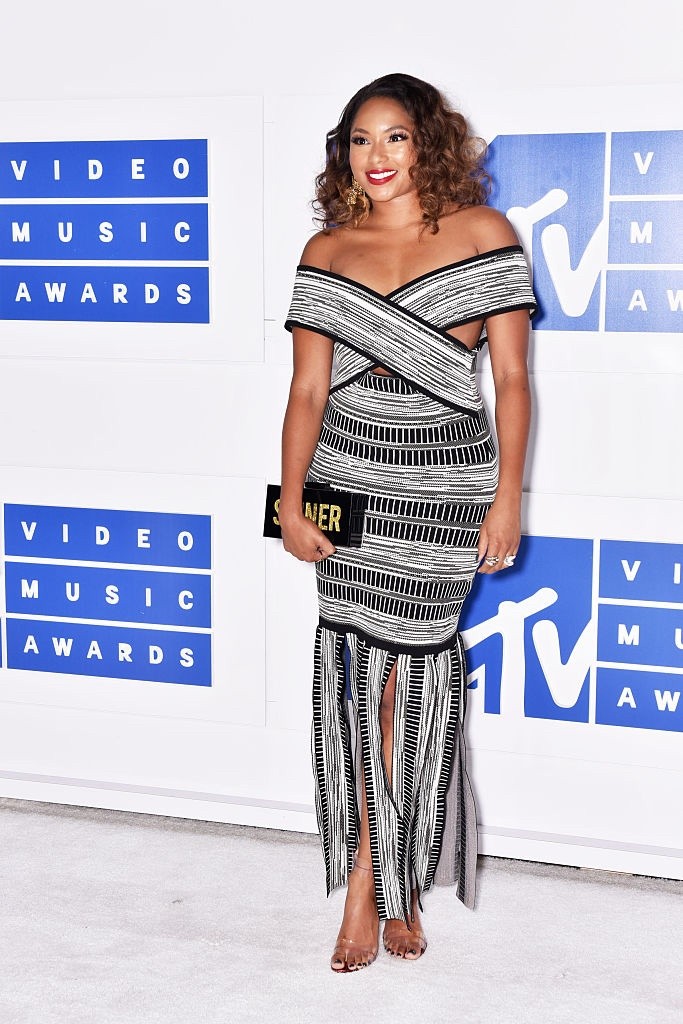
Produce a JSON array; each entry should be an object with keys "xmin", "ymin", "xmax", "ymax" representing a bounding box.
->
[
  {"xmin": 382, "ymin": 887, "xmax": 427, "ymax": 962},
  {"xmin": 331, "ymin": 857, "xmax": 380, "ymax": 973}
]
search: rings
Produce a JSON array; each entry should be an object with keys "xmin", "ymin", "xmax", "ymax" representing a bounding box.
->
[
  {"xmin": 504, "ymin": 556, "xmax": 516, "ymax": 566},
  {"xmin": 485, "ymin": 557, "xmax": 499, "ymax": 566},
  {"xmin": 315, "ymin": 550, "xmax": 323, "ymax": 564}
]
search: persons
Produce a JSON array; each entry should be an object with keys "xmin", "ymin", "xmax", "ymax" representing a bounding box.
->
[{"xmin": 279, "ymin": 73, "xmax": 539, "ymax": 974}]
